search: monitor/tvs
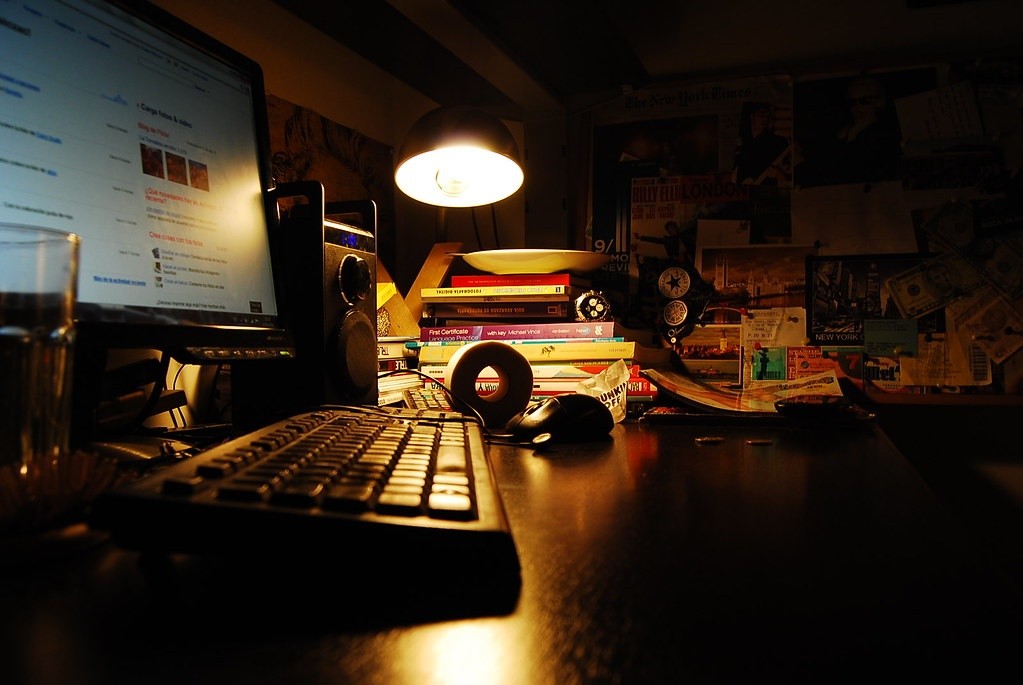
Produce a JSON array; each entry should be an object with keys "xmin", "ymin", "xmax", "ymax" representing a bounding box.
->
[{"xmin": 0, "ymin": 0, "xmax": 296, "ymax": 468}]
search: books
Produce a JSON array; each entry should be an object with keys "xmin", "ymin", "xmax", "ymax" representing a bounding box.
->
[{"xmin": 376, "ymin": 272, "xmax": 661, "ymax": 404}]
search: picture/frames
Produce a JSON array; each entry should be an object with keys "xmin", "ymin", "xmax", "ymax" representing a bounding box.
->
[{"xmin": 803, "ymin": 251, "xmax": 947, "ymax": 346}]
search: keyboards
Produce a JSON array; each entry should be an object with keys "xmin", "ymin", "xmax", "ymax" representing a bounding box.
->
[{"xmin": 106, "ymin": 403, "xmax": 521, "ymax": 579}]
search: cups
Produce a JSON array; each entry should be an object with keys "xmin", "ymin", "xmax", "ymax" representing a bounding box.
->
[{"xmin": 0, "ymin": 220, "xmax": 84, "ymax": 539}]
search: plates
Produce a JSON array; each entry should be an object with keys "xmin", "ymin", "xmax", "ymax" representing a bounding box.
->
[{"xmin": 460, "ymin": 247, "xmax": 609, "ymax": 274}]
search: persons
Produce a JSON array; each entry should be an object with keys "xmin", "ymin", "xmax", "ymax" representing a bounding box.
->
[{"xmin": 633, "ymin": 221, "xmax": 697, "ymax": 263}]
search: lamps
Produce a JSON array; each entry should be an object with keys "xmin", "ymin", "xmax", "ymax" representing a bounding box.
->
[{"xmin": 392, "ymin": 102, "xmax": 526, "ymax": 244}]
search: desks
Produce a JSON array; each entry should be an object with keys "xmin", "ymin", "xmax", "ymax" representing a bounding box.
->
[
  {"xmin": 0, "ymin": 405, "xmax": 1023, "ymax": 685},
  {"xmin": 853, "ymin": 389, "xmax": 1023, "ymax": 469}
]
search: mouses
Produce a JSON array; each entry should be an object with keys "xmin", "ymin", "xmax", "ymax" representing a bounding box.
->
[{"xmin": 505, "ymin": 394, "xmax": 614, "ymax": 447}]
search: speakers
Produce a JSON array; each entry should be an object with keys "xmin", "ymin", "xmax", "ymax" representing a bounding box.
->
[{"xmin": 228, "ymin": 178, "xmax": 378, "ymax": 434}]
